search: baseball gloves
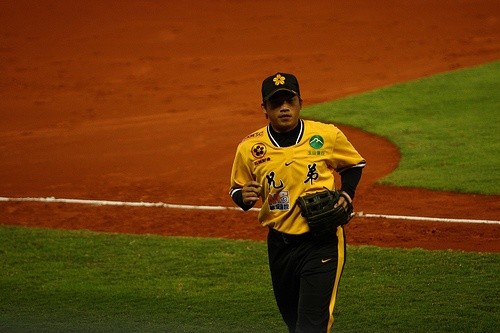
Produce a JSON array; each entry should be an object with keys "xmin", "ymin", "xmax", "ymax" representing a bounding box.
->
[{"xmin": 297, "ymin": 186, "xmax": 354, "ymax": 231}]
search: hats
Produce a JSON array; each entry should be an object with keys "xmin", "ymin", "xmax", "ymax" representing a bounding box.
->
[{"xmin": 261, "ymin": 72, "xmax": 300, "ymax": 103}]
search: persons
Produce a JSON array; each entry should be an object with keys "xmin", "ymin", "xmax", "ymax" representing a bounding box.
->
[{"xmin": 228, "ymin": 71, "xmax": 367, "ymax": 332}]
